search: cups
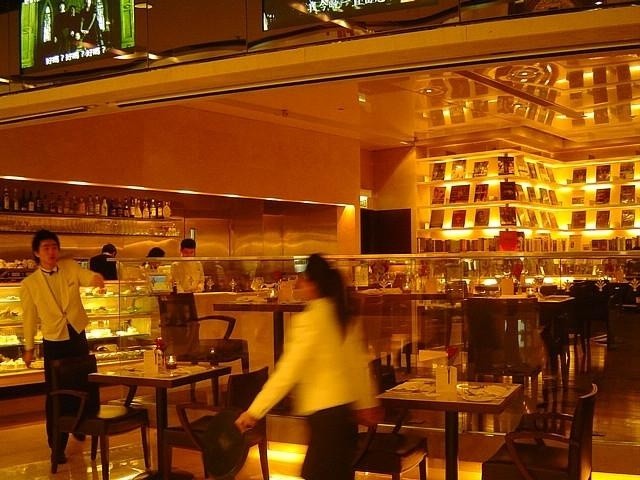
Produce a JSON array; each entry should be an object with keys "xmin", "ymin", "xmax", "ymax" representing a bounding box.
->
[{"xmin": 166, "ymin": 356, "xmax": 177, "ymax": 370}]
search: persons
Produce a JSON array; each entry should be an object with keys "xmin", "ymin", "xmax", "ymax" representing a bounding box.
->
[
  {"xmin": 141, "ymin": 247, "xmax": 164, "ymax": 269},
  {"xmin": 52, "ymin": 0, "xmax": 99, "ymax": 56},
  {"xmin": 235, "ymin": 252, "xmax": 378, "ymax": 480},
  {"xmin": 169, "ymin": 240, "xmax": 205, "ymax": 293},
  {"xmin": 87, "ymin": 244, "xmax": 125, "ymax": 280},
  {"xmin": 20, "ymin": 228, "xmax": 104, "ymax": 464}
]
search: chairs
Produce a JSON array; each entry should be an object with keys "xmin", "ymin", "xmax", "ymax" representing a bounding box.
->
[
  {"xmin": 354, "ymin": 407, "xmax": 428, "ymax": 480},
  {"xmin": 47, "ymin": 292, "xmax": 269, "ymax": 480},
  {"xmin": 481, "ymin": 384, "xmax": 598, "ymax": 480},
  {"xmin": 382, "ymin": 281, "xmax": 612, "ymax": 434}
]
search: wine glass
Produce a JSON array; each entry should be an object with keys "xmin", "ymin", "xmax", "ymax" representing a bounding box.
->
[
  {"xmin": 532, "ymin": 275, "xmax": 545, "ymax": 299},
  {"xmin": 249, "ymin": 275, "xmax": 294, "ymax": 303},
  {"xmin": 1, "ymin": 215, "xmax": 176, "ymax": 236},
  {"xmin": 377, "ymin": 272, "xmax": 429, "ymax": 293}
]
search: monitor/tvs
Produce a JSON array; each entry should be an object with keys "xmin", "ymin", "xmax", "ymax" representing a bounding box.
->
[
  {"xmin": 262, "ymin": 0, "xmax": 439, "ymax": 32},
  {"xmin": 18, "ymin": 0, "xmax": 135, "ymax": 73}
]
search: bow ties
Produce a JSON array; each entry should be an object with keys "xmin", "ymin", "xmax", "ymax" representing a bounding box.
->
[{"xmin": 41, "ymin": 269, "xmax": 57, "ymax": 277}]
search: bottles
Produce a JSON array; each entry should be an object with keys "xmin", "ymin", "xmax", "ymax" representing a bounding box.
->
[
  {"xmin": 0, "ymin": 183, "xmax": 172, "ymax": 221},
  {"xmin": 153, "ymin": 339, "xmax": 164, "ymax": 368}
]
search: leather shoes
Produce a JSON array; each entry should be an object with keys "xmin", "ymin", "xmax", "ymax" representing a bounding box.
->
[
  {"xmin": 71, "ymin": 431, "xmax": 86, "ymax": 442},
  {"xmin": 52, "ymin": 449, "xmax": 66, "ymax": 464}
]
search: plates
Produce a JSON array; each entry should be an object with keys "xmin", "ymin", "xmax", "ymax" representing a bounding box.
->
[
  {"xmin": 457, "ymin": 383, "xmax": 469, "ymax": 389},
  {"xmin": 390, "ymin": 378, "xmax": 435, "ymax": 395},
  {"xmin": 292, "ymin": 300, "xmax": 306, "ymax": 304},
  {"xmin": 464, "ymin": 394, "xmax": 498, "ymax": 402}
]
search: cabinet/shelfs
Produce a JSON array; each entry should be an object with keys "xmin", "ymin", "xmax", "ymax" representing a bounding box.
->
[
  {"xmin": 0, "ymin": 210, "xmax": 182, "ymax": 240},
  {"xmin": 0, "ymin": 281, "xmax": 160, "ymax": 390},
  {"xmin": 416, "ymin": 152, "xmax": 639, "ymax": 254}
]
